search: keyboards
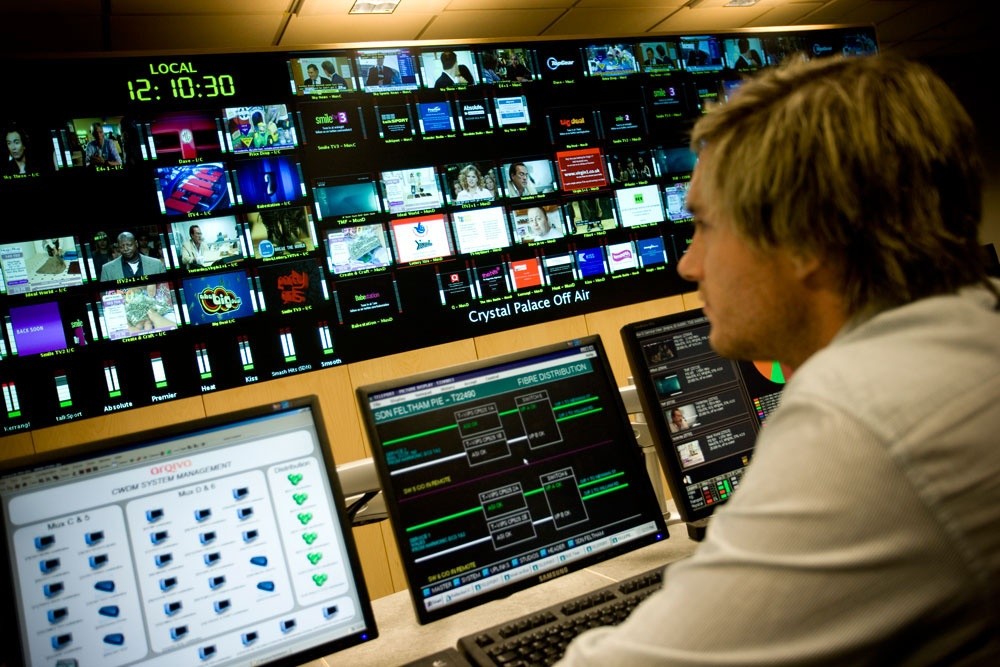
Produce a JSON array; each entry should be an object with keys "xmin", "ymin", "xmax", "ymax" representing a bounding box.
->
[{"xmin": 456, "ymin": 562, "xmax": 680, "ymax": 667}]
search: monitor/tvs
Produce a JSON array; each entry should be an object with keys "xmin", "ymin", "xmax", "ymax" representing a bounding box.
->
[
  {"xmin": 620, "ymin": 306, "xmax": 793, "ymax": 522},
  {"xmin": 0, "ymin": 394, "xmax": 379, "ymax": 667},
  {"xmin": 355, "ymin": 334, "xmax": 670, "ymax": 626},
  {"xmin": 0, "ymin": 23, "xmax": 883, "ymax": 439}
]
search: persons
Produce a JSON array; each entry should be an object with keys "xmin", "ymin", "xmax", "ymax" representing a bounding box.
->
[
  {"xmin": 92, "ymin": 228, "xmax": 167, "ymax": 283},
  {"xmin": 366, "ymin": 53, "xmax": 393, "ymax": 86},
  {"xmin": 432, "ymin": 51, "xmax": 533, "ymax": 88},
  {"xmin": 178, "ymin": 224, "xmax": 212, "ymax": 267},
  {"xmin": 665, "ymin": 404, "xmax": 699, "ymax": 433},
  {"xmin": 643, "ymin": 43, "xmax": 675, "ymax": 70},
  {"xmin": 526, "ymin": 205, "xmax": 565, "ymax": 242},
  {"xmin": 83, "ymin": 121, "xmax": 122, "ymax": 167},
  {"xmin": 1, "ymin": 125, "xmax": 48, "ymax": 176},
  {"xmin": 613, "ymin": 150, "xmax": 652, "ymax": 185},
  {"xmin": 731, "ymin": 37, "xmax": 764, "ymax": 69},
  {"xmin": 590, "ymin": 45, "xmax": 636, "ymax": 77},
  {"xmin": 302, "ymin": 60, "xmax": 348, "ymax": 92},
  {"xmin": 686, "ymin": 38, "xmax": 708, "ymax": 66},
  {"xmin": 507, "ymin": 161, "xmax": 541, "ymax": 199},
  {"xmin": 449, "ymin": 164, "xmax": 502, "ymax": 203},
  {"xmin": 545, "ymin": 50, "xmax": 1000, "ymax": 667}
]
